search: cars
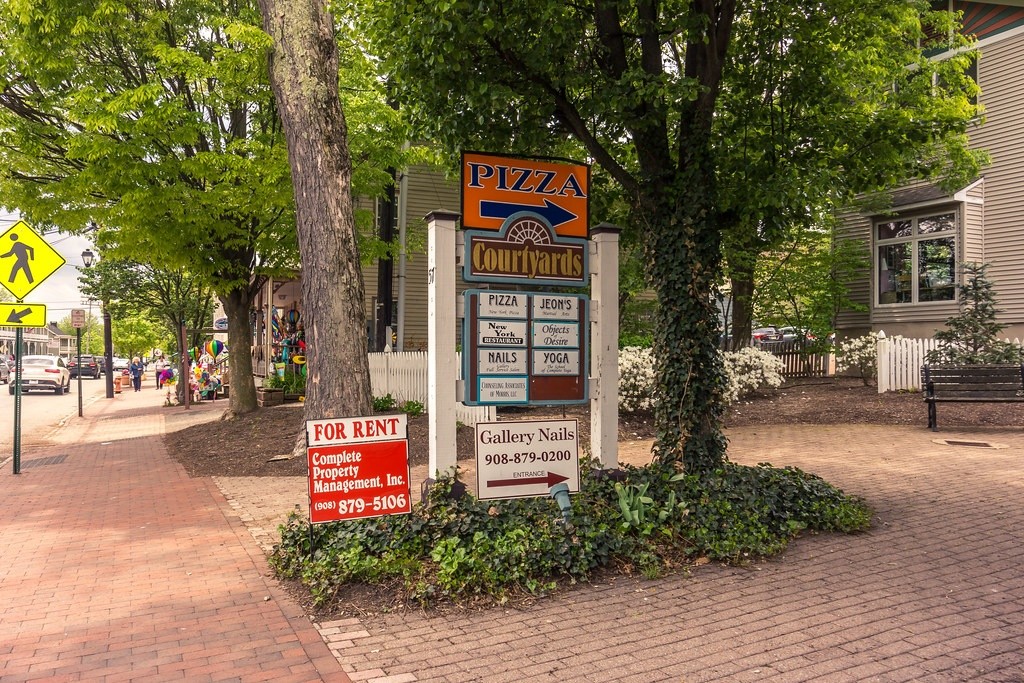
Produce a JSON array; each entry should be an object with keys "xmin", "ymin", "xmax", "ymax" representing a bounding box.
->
[
  {"xmin": 95, "ymin": 356, "xmax": 131, "ymax": 375},
  {"xmin": 7, "ymin": 354, "xmax": 72, "ymax": 396},
  {"xmin": 0, "ymin": 356, "xmax": 11, "ymax": 384},
  {"xmin": 719, "ymin": 325, "xmax": 836, "ymax": 353}
]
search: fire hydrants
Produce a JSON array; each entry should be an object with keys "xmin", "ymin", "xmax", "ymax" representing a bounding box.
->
[{"xmin": 113, "ymin": 376, "xmax": 123, "ymax": 393}]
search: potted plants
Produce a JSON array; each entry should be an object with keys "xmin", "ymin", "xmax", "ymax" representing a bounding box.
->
[{"xmin": 258, "ymin": 369, "xmax": 285, "ymax": 392}]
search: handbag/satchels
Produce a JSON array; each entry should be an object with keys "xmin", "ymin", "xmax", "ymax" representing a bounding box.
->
[
  {"xmin": 130, "ymin": 371, "xmax": 134, "ymax": 379},
  {"xmin": 141, "ymin": 374, "xmax": 146, "ymax": 381}
]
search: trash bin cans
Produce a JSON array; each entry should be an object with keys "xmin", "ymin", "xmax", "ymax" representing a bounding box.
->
[{"xmin": 123, "ymin": 373, "xmax": 129, "ymax": 385}]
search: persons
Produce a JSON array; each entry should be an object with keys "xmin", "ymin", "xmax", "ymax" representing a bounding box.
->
[
  {"xmin": 7, "ymin": 355, "xmax": 15, "ymax": 374},
  {"xmin": 128, "ymin": 357, "xmax": 143, "ymax": 392},
  {"xmin": 154, "ymin": 357, "xmax": 165, "ymax": 390}
]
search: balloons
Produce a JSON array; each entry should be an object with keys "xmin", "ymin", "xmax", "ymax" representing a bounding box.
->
[
  {"xmin": 188, "ymin": 340, "xmax": 223, "ymax": 390},
  {"xmin": 272, "ymin": 309, "xmax": 301, "ymax": 332}
]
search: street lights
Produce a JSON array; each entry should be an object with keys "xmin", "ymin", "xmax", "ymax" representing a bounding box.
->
[{"xmin": 80, "ymin": 249, "xmax": 95, "ymax": 356}]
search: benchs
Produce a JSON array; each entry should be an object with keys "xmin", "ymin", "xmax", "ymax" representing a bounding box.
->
[{"xmin": 920, "ymin": 362, "xmax": 1024, "ymax": 434}]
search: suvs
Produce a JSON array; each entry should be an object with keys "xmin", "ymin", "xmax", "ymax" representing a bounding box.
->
[{"xmin": 67, "ymin": 355, "xmax": 101, "ymax": 379}]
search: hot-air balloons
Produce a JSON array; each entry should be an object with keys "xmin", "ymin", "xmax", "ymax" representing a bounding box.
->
[
  {"xmin": 188, "ymin": 346, "xmax": 202, "ymax": 363},
  {"xmin": 205, "ymin": 340, "xmax": 223, "ymax": 364},
  {"xmin": 287, "ymin": 310, "xmax": 300, "ymax": 330}
]
